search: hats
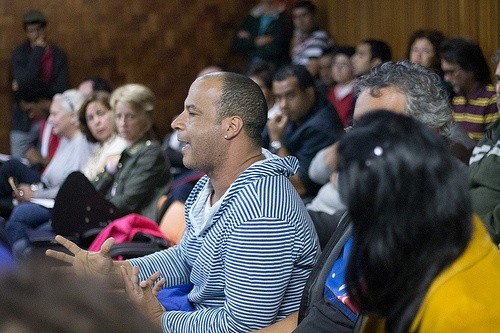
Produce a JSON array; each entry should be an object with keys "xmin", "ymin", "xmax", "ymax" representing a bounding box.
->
[{"xmin": 23, "ymin": 10, "xmax": 48, "ymax": 25}]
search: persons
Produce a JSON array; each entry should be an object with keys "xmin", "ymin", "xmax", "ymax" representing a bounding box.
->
[
  {"xmin": 0, "ymin": 30, "xmax": 500, "ymax": 333},
  {"xmin": 9, "ymin": 10, "xmax": 71, "ymax": 169},
  {"xmin": 231, "ymin": 0, "xmax": 294, "ymax": 75},
  {"xmin": 45, "ymin": 72, "xmax": 323, "ymax": 333},
  {"xmin": 289, "ymin": 0, "xmax": 335, "ymax": 88}
]
